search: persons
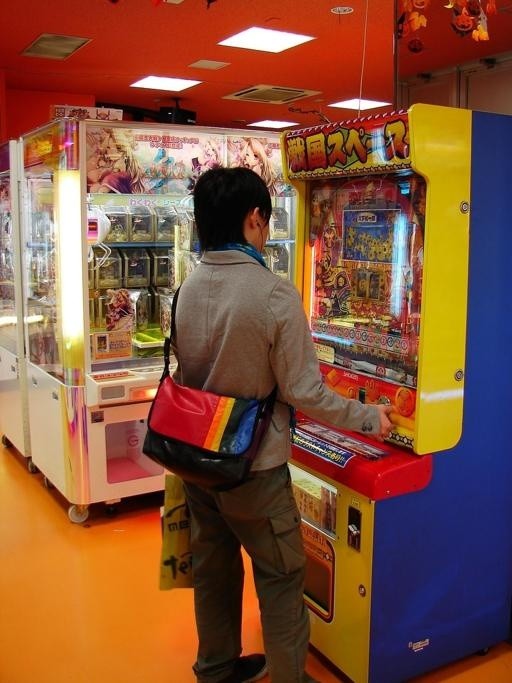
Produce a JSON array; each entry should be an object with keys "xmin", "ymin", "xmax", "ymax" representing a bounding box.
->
[{"xmin": 162, "ymin": 165, "xmax": 401, "ymax": 682}]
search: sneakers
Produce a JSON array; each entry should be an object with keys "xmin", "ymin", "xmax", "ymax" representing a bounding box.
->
[{"xmin": 219, "ymin": 654, "xmax": 269, "ymax": 682}]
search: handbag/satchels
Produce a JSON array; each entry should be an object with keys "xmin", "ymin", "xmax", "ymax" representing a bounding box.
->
[{"xmin": 141, "ymin": 377, "xmax": 273, "ymax": 493}]
